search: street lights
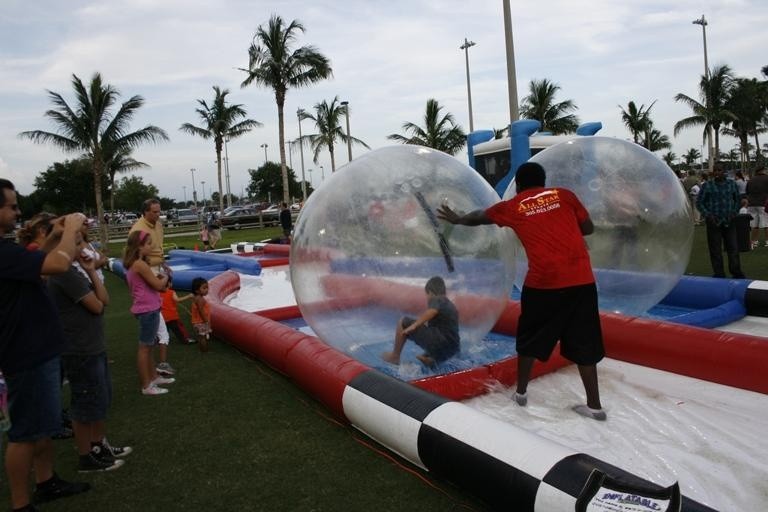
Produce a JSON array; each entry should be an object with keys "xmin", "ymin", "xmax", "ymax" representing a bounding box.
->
[
  {"xmin": 217, "ymin": 131, "xmax": 233, "ymax": 206},
  {"xmin": 261, "ymin": 142, "xmax": 274, "ymax": 203},
  {"xmin": 460, "ymin": 37, "xmax": 476, "ymax": 134},
  {"xmin": 284, "ymin": 140, "xmax": 295, "ymax": 205},
  {"xmin": 296, "ymin": 106, "xmax": 310, "ymax": 204},
  {"xmin": 341, "ymin": 99, "xmax": 354, "ymax": 163},
  {"xmin": 318, "ymin": 166, "xmax": 325, "ymax": 181},
  {"xmin": 692, "ymin": 13, "xmax": 715, "ymax": 179},
  {"xmin": 183, "ymin": 157, "xmax": 231, "ymax": 208},
  {"xmin": 306, "ymin": 168, "xmax": 313, "ymax": 188}
]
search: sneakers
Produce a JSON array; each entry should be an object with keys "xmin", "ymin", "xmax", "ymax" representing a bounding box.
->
[
  {"xmin": 52, "ymin": 408, "xmax": 75, "ymax": 441},
  {"xmin": 90, "ymin": 436, "xmax": 125, "ymax": 467},
  {"xmin": 155, "ymin": 361, "xmax": 178, "ymax": 375},
  {"xmin": 76, "ymin": 452, "xmax": 120, "ymax": 475},
  {"xmin": 141, "ymin": 382, "xmax": 169, "ymax": 395},
  {"xmin": 152, "ymin": 375, "xmax": 175, "ymax": 385},
  {"xmin": 32, "ymin": 473, "xmax": 93, "ymax": 502},
  {"xmin": 100, "ymin": 436, "xmax": 134, "ymax": 458}
]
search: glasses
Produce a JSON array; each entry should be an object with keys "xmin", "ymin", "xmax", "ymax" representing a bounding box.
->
[{"xmin": 1, "ymin": 202, "xmax": 19, "ymax": 210}]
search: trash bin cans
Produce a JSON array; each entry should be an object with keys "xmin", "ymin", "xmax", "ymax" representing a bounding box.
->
[{"xmin": 734, "ymin": 213, "xmax": 754, "ymax": 252}]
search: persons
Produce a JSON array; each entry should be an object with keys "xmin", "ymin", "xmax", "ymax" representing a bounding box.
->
[
  {"xmin": 603, "ymin": 181, "xmax": 652, "ymax": 272},
  {"xmin": 0, "ymin": 178, "xmax": 133, "ymax": 512},
  {"xmin": 299, "ymin": 197, "xmax": 305, "ymax": 210},
  {"xmin": 379, "ymin": 277, "xmax": 459, "ymax": 367},
  {"xmin": 435, "ymin": 162, "xmax": 608, "ymax": 419},
  {"xmin": 122, "ymin": 199, "xmax": 221, "ymax": 396},
  {"xmin": 279, "ymin": 202, "xmax": 293, "ymax": 241},
  {"xmin": 675, "ymin": 161, "xmax": 768, "ymax": 278}
]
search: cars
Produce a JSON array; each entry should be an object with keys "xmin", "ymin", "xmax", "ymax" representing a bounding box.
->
[{"xmin": 86, "ymin": 201, "xmax": 303, "ymax": 232}]
